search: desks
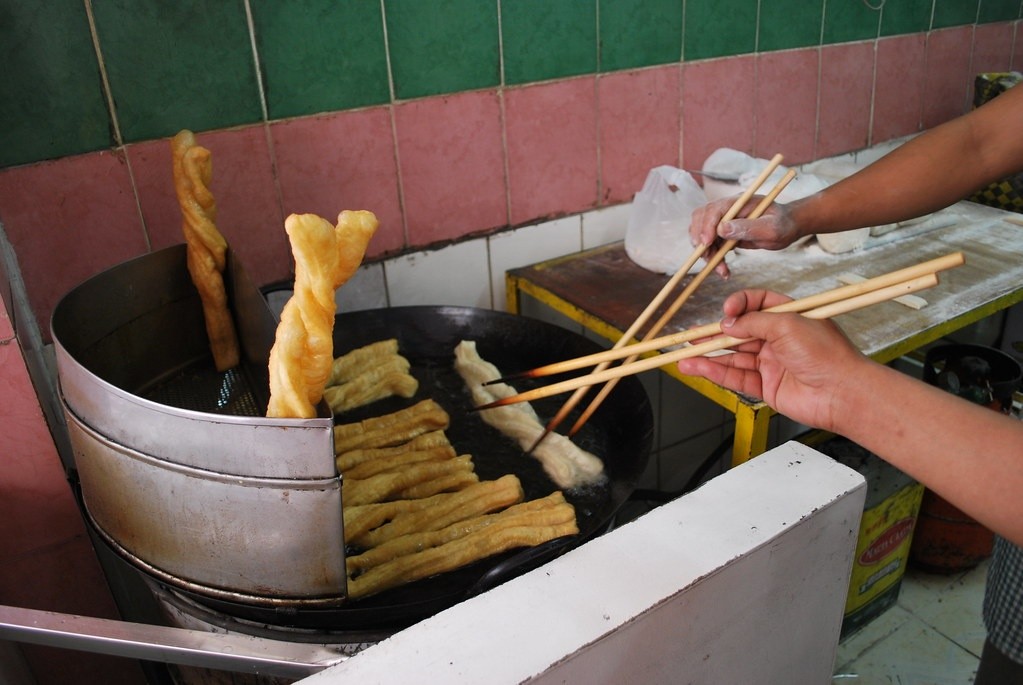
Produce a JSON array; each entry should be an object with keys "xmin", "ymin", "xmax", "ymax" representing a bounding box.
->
[{"xmin": 502, "ymin": 160, "xmax": 1023, "ymax": 471}]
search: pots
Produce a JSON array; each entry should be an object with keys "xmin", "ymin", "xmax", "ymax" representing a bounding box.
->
[{"xmin": 129, "ymin": 306, "xmax": 653, "ymax": 631}]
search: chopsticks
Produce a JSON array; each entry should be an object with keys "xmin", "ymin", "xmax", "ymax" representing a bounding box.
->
[
  {"xmin": 467, "ymin": 252, "xmax": 968, "ymax": 413},
  {"xmin": 529, "ymin": 153, "xmax": 796, "ymax": 451}
]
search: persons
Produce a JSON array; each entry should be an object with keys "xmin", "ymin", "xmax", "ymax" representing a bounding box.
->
[
  {"xmin": 678, "ymin": 289, "xmax": 1023, "ymax": 547},
  {"xmin": 688, "ymin": 79, "xmax": 1023, "ymax": 685}
]
